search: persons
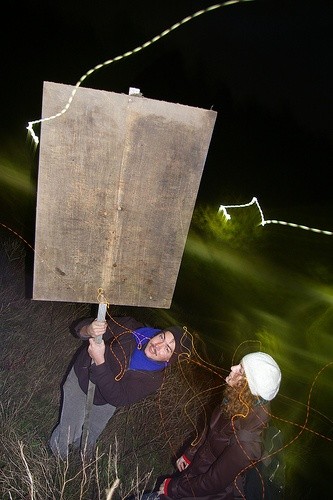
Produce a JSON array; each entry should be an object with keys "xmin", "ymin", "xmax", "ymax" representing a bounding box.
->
[
  {"xmin": 126, "ymin": 351, "xmax": 281, "ymax": 500},
  {"xmin": 51, "ymin": 316, "xmax": 195, "ymax": 464}
]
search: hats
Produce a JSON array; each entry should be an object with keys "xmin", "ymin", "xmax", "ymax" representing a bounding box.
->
[
  {"xmin": 162, "ymin": 325, "xmax": 190, "ymax": 364},
  {"xmin": 241, "ymin": 350, "xmax": 283, "ymax": 401}
]
